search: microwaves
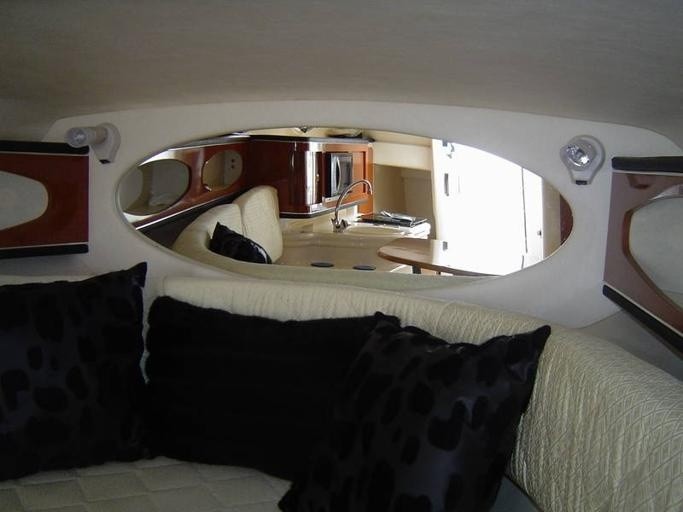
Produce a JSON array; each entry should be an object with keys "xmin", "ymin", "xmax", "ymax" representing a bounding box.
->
[{"xmin": 326, "ymin": 150, "xmax": 352, "ymax": 203}]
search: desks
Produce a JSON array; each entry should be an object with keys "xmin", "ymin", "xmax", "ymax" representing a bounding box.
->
[{"xmin": 375, "ymin": 230, "xmax": 536, "ymax": 276}]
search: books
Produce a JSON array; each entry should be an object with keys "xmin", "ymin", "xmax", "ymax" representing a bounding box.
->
[{"xmin": 354, "ymin": 209, "xmax": 429, "ymax": 228}]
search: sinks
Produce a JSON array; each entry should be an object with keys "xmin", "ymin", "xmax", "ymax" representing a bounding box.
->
[{"xmin": 343, "ymin": 226, "xmax": 404, "ymax": 236}]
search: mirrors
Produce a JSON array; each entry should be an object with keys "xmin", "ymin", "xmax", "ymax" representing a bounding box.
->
[{"xmin": 113, "ymin": 124, "xmax": 573, "ymax": 294}]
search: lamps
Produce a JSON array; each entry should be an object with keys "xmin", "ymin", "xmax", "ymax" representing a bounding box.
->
[
  {"xmin": 559, "ymin": 133, "xmax": 605, "ymax": 185},
  {"xmin": 64, "ymin": 120, "xmax": 120, "ymax": 163}
]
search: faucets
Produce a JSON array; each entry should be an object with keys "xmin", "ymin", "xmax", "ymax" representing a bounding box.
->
[{"xmin": 331, "ymin": 180, "xmax": 372, "ymax": 232}]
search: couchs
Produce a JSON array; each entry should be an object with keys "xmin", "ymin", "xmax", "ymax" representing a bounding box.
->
[
  {"xmin": 172, "ymin": 182, "xmax": 287, "ymax": 265},
  {"xmin": 0, "ymin": 268, "xmax": 681, "ymax": 511}
]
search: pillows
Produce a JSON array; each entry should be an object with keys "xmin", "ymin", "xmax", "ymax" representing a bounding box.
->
[
  {"xmin": 0, "ymin": 259, "xmax": 152, "ymax": 481},
  {"xmin": 272, "ymin": 315, "xmax": 553, "ymax": 512},
  {"xmin": 136, "ymin": 294, "xmax": 401, "ymax": 482},
  {"xmin": 210, "ymin": 219, "xmax": 270, "ymax": 263}
]
